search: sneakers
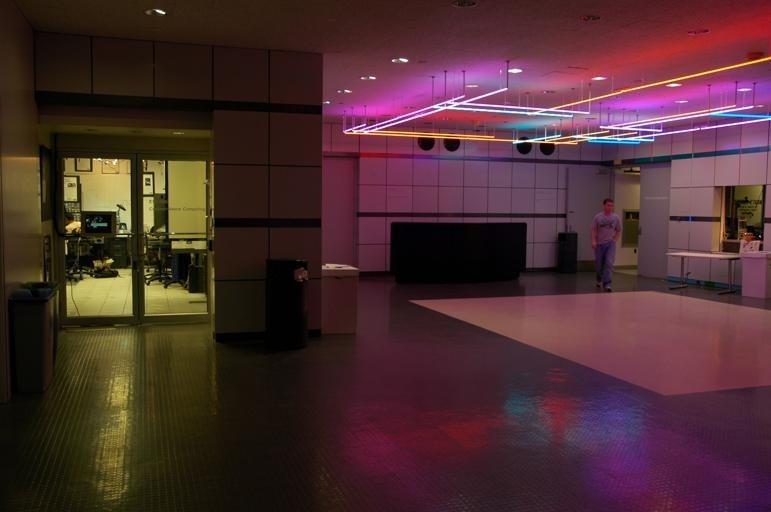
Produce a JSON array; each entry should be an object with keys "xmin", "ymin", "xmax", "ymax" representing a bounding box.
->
[{"xmin": 596, "ymin": 280, "xmax": 612, "ymax": 292}]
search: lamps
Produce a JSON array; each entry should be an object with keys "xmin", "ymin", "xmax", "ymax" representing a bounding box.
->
[{"xmin": 341, "ymin": 54, "xmax": 771, "ymax": 145}]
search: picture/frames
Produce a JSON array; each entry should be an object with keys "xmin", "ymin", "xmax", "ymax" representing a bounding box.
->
[
  {"xmin": 142, "ymin": 172, "xmax": 155, "ymax": 196},
  {"xmin": 75, "ymin": 158, "xmax": 92, "ymax": 172},
  {"xmin": 101, "ymin": 159, "xmax": 119, "ymax": 174},
  {"xmin": 127, "ymin": 159, "xmax": 147, "ymax": 174},
  {"xmin": 64, "ymin": 176, "xmax": 80, "ymax": 202}
]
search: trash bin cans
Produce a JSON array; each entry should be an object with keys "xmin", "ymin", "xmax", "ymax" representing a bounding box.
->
[
  {"xmin": 264, "ymin": 259, "xmax": 308, "ymax": 352},
  {"xmin": 558, "ymin": 232, "xmax": 577, "ymax": 274},
  {"xmin": 8, "ymin": 281, "xmax": 62, "ymax": 393}
]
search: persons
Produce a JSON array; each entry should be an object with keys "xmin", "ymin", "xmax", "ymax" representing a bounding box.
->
[
  {"xmin": 65, "ymin": 211, "xmax": 83, "ymax": 236},
  {"xmin": 590, "ymin": 198, "xmax": 624, "ymax": 291}
]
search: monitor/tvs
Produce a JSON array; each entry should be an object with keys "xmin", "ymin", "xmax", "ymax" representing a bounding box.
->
[{"xmin": 79, "ymin": 211, "xmax": 117, "ymax": 236}]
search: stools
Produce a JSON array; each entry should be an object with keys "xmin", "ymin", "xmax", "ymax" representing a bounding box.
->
[{"xmin": 143, "ymin": 232, "xmax": 185, "ymax": 288}]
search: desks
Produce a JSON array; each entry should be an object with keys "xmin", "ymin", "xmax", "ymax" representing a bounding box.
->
[{"xmin": 666, "ymin": 248, "xmax": 741, "ymax": 295}]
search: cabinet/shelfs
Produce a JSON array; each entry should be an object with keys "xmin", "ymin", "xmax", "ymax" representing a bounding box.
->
[{"xmin": 321, "ymin": 264, "xmax": 359, "ymax": 334}]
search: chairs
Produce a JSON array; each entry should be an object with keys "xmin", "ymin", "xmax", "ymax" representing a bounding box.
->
[{"xmin": 65, "ymin": 226, "xmax": 95, "ymax": 281}]
